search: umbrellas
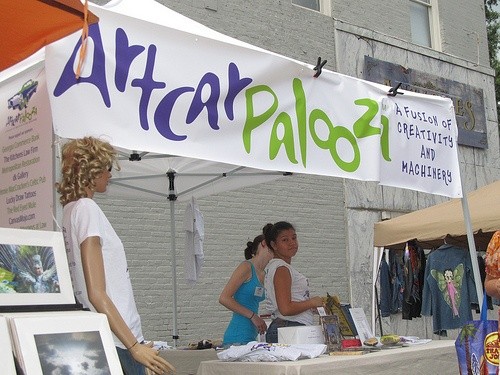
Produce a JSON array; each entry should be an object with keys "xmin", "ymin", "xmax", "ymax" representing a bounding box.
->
[{"xmin": 0, "ymin": 0, "xmax": 99, "ymax": 73}]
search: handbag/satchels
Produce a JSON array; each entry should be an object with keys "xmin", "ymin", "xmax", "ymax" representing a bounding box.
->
[{"xmin": 455, "ymin": 289, "xmax": 500, "ymax": 375}]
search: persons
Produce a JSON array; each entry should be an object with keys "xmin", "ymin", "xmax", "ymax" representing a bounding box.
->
[
  {"xmin": 55, "ymin": 136, "xmax": 174, "ymax": 375},
  {"xmin": 262, "ymin": 222, "xmax": 324, "ymax": 344},
  {"xmin": 218, "ymin": 234, "xmax": 274, "ymax": 345}
]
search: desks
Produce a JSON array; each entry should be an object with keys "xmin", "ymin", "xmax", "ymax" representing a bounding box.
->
[
  {"xmin": 196, "ymin": 340, "xmax": 461, "ymax": 375},
  {"xmin": 158, "ymin": 349, "xmax": 218, "ymax": 375}
]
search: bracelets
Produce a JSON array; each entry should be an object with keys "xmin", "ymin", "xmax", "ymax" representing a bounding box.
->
[{"xmin": 250, "ymin": 312, "xmax": 254, "ymax": 319}]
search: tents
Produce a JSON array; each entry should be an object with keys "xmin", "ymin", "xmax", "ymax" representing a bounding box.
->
[
  {"xmin": 370, "ymin": 163, "xmax": 499, "ymax": 375},
  {"xmin": 0, "ymin": 0, "xmax": 486, "ymax": 351}
]
